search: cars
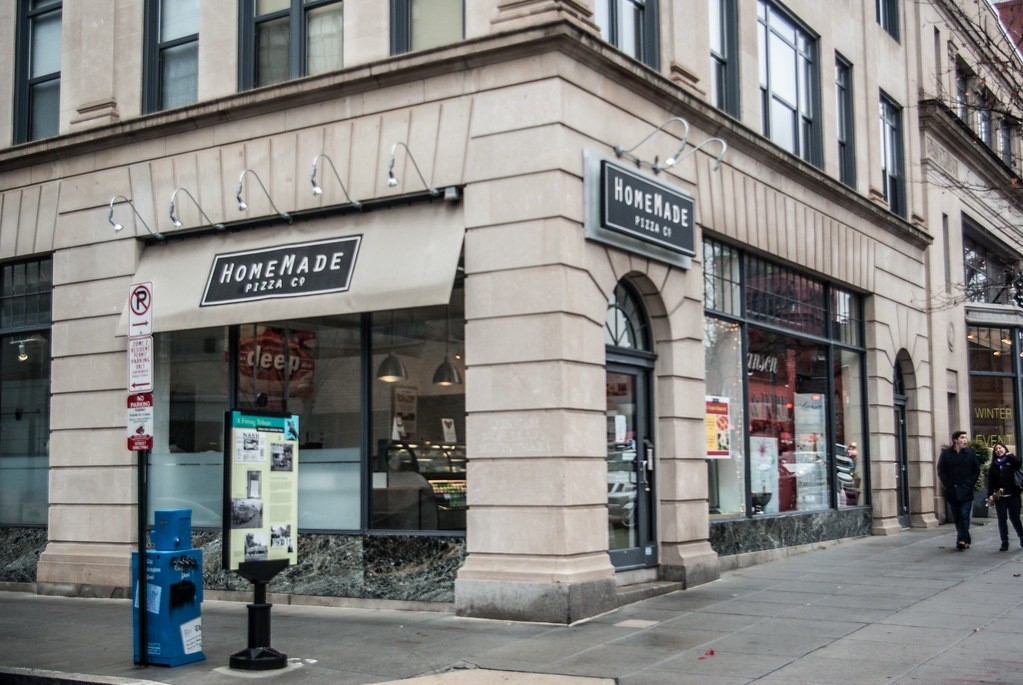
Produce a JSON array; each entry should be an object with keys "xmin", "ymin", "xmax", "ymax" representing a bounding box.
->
[
  {"xmin": 751, "ymin": 439, "xmax": 857, "ymax": 512},
  {"xmin": 605, "ymin": 439, "xmax": 638, "ymax": 524}
]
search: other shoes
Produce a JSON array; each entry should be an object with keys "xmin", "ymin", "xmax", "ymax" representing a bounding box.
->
[{"xmin": 956, "ymin": 540, "xmax": 969, "ymax": 548}]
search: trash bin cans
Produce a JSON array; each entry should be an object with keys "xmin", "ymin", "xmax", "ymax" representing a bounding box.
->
[{"xmin": 131, "ymin": 509, "xmax": 207, "ymax": 666}]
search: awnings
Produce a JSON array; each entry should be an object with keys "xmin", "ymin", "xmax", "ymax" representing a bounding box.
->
[{"xmin": 115, "ymin": 199, "xmax": 465, "ymax": 338}]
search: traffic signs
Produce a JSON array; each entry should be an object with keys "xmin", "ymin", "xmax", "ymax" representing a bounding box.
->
[
  {"xmin": 127, "ymin": 280, "xmax": 154, "ymax": 340},
  {"xmin": 126, "ymin": 336, "xmax": 154, "ymax": 393}
]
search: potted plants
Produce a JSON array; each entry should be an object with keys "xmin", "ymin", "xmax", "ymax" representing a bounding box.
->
[{"xmin": 943, "ymin": 439, "xmax": 992, "ymax": 522}]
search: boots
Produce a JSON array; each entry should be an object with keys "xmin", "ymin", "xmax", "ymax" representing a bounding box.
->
[
  {"xmin": 1020, "ymin": 538, "xmax": 1023, "ymax": 547},
  {"xmin": 1000, "ymin": 540, "xmax": 1008, "ymax": 551}
]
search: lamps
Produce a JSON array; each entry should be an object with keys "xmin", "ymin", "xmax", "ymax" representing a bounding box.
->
[
  {"xmin": 309, "ymin": 153, "xmax": 360, "ymax": 207},
  {"xmin": 169, "ymin": 186, "xmax": 223, "ymax": 229},
  {"xmin": 388, "ymin": 141, "xmax": 438, "ymax": 194},
  {"xmin": 653, "ymin": 137, "xmax": 728, "ymax": 173},
  {"xmin": 17, "ymin": 341, "xmax": 27, "ymax": 360},
  {"xmin": 615, "ymin": 116, "xmax": 689, "ymax": 167},
  {"xmin": 433, "ymin": 304, "xmax": 462, "ymax": 386},
  {"xmin": 108, "ymin": 193, "xmax": 162, "ymax": 240},
  {"xmin": 237, "ymin": 169, "xmax": 285, "ymax": 219},
  {"xmin": 375, "ymin": 310, "xmax": 408, "ymax": 385}
]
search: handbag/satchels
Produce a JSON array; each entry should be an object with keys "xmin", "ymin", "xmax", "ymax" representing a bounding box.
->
[{"xmin": 1013, "ymin": 468, "xmax": 1023, "ymax": 492}]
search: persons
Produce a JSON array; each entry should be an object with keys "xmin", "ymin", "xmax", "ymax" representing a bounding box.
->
[
  {"xmin": 937, "ymin": 430, "xmax": 980, "ymax": 550},
  {"xmin": 987, "ymin": 443, "xmax": 1023, "ymax": 552}
]
